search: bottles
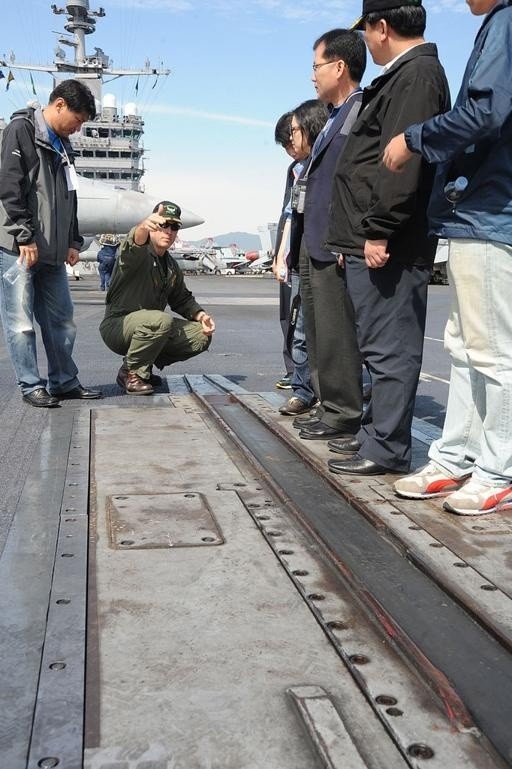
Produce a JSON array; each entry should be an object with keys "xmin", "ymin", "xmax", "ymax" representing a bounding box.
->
[
  {"xmin": 442, "ymin": 176, "xmax": 468, "ymax": 205},
  {"xmin": 2, "ymin": 256, "xmax": 29, "ymax": 286},
  {"xmin": 279, "ymin": 269, "xmax": 291, "ymax": 288}
]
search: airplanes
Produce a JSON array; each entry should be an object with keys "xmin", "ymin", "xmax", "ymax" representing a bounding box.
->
[{"xmin": 64, "ymin": 171, "xmax": 275, "ymax": 282}]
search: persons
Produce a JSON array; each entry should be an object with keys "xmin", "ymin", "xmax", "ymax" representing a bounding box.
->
[
  {"xmin": 272, "ymin": 112, "xmax": 309, "ymax": 388},
  {"xmin": 381, "ymin": 0, "xmax": 512, "ymax": 516},
  {"xmin": 99, "ymin": 201, "xmax": 215, "ymax": 396},
  {"xmin": 0, "ymin": 79, "xmax": 103, "ymax": 407},
  {"xmin": 97, "ymin": 240, "xmax": 121, "ymax": 291},
  {"xmin": 278, "ymin": 100, "xmax": 331, "ymax": 416},
  {"xmin": 327, "ymin": 1, "xmax": 452, "ymax": 475},
  {"xmin": 292, "ymin": 28, "xmax": 362, "ymax": 441}
]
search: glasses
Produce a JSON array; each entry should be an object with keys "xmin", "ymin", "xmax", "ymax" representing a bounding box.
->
[
  {"xmin": 157, "ymin": 220, "xmax": 181, "ymax": 231},
  {"xmin": 313, "ymin": 58, "xmax": 342, "ymax": 71},
  {"xmin": 290, "ymin": 126, "xmax": 307, "ymax": 135}
]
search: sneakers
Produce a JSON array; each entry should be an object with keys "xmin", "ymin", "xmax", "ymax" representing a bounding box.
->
[
  {"xmin": 275, "ymin": 376, "xmax": 293, "ymax": 389},
  {"xmin": 278, "ymin": 395, "xmax": 311, "ymax": 415},
  {"xmin": 441, "ymin": 477, "xmax": 512, "ymax": 517},
  {"xmin": 391, "ymin": 459, "xmax": 472, "ymax": 500}
]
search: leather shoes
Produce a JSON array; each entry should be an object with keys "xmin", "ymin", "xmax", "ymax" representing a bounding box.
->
[
  {"xmin": 51, "ymin": 385, "xmax": 106, "ymax": 400},
  {"xmin": 117, "ymin": 364, "xmax": 155, "ymax": 396},
  {"xmin": 326, "ymin": 435, "xmax": 364, "ymax": 457},
  {"xmin": 292, "ymin": 416, "xmax": 320, "ymax": 430},
  {"xmin": 326, "ymin": 450, "xmax": 394, "ymax": 476},
  {"xmin": 298, "ymin": 420, "xmax": 357, "ymax": 441},
  {"xmin": 22, "ymin": 387, "xmax": 58, "ymax": 407}
]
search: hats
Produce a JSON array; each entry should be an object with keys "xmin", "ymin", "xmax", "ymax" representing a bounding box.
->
[
  {"xmin": 348, "ymin": 0, "xmax": 423, "ymax": 32},
  {"xmin": 152, "ymin": 201, "xmax": 183, "ymax": 224}
]
